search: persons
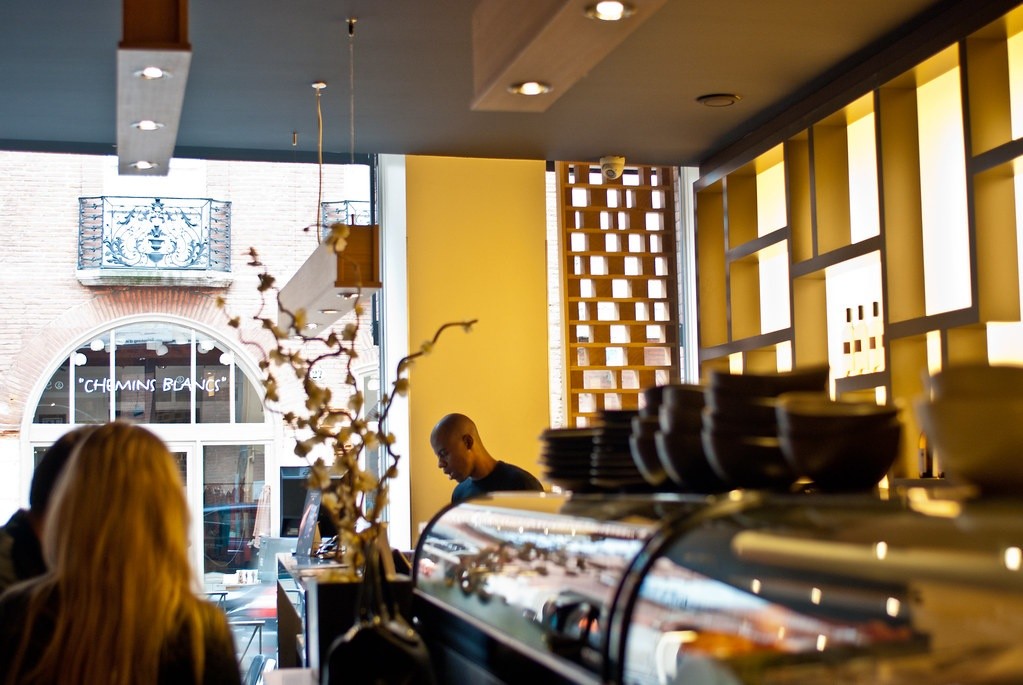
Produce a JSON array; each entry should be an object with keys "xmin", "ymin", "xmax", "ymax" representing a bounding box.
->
[
  {"xmin": 0, "ymin": 418, "xmax": 245, "ymax": 685},
  {"xmin": 430, "ymin": 413, "xmax": 544, "ymax": 503}
]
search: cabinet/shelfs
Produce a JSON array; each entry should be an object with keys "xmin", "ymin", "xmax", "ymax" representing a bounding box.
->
[
  {"xmin": 557, "ymin": 160, "xmax": 681, "ymax": 429},
  {"xmin": 693, "ymin": 1, "xmax": 1023, "ymax": 502}
]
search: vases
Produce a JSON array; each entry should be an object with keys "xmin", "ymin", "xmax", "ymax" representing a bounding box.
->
[{"xmin": 326, "ymin": 522, "xmax": 434, "ymax": 685}]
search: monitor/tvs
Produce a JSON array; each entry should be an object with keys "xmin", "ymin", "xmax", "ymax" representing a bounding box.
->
[{"xmin": 279, "ymin": 467, "xmax": 352, "ymax": 557}]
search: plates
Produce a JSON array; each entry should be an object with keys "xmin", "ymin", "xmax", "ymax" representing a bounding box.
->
[
  {"xmin": 539, "ymin": 427, "xmax": 591, "ymax": 493},
  {"xmin": 591, "ymin": 409, "xmax": 639, "ymax": 493}
]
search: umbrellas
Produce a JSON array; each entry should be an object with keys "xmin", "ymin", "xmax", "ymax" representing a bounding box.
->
[{"xmin": 252, "ymin": 485, "xmax": 272, "ymax": 547}]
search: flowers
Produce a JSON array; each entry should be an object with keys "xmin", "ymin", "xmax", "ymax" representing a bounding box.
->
[{"xmin": 206, "ymin": 220, "xmax": 478, "ymax": 579}]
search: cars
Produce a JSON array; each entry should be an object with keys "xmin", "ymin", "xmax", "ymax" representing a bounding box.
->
[{"xmin": 202, "ymin": 504, "xmax": 262, "ymax": 572}]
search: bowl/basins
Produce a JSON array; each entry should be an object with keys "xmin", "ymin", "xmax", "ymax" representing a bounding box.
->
[
  {"xmin": 654, "ymin": 387, "xmax": 717, "ymax": 491},
  {"xmin": 701, "ymin": 366, "xmax": 829, "ymax": 493},
  {"xmin": 629, "ymin": 386, "xmax": 675, "ymax": 491},
  {"xmin": 779, "ymin": 400, "xmax": 902, "ymax": 495}
]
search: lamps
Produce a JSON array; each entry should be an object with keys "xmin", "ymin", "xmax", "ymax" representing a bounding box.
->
[
  {"xmin": 468, "ymin": 0, "xmax": 670, "ymax": 112},
  {"xmin": 277, "ymin": 18, "xmax": 381, "ymax": 338},
  {"xmin": 697, "ymin": 94, "xmax": 740, "ymax": 110},
  {"xmin": 117, "ymin": 0, "xmax": 193, "ymax": 175}
]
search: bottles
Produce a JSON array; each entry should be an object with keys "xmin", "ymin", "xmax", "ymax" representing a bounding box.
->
[
  {"xmin": 842, "ymin": 308, "xmax": 854, "ymax": 378},
  {"xmin": 853, "ymin": 305, "xmax": 869, "ymax": 375},
  {"xmin": 869, "ymin": 302, "xmax": 882, "ymax": 373},
  {"xmin": 918, "ymin": 428, "xmax": 933, "ymax": 477}
]
